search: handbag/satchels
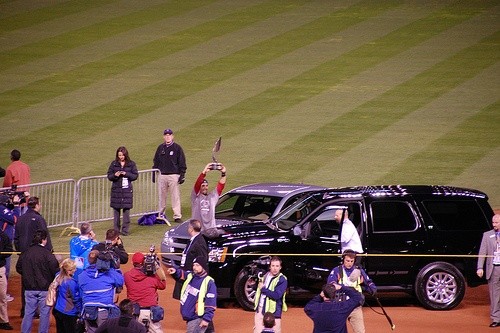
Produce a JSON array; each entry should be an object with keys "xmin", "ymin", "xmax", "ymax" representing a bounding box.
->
[
  {"xmin": 46, "ymin": 275, "xmax": 61, "ymax": 307},
  {"xmin": 150, "ymin": 306, "xmax": 164, "ymax": 323},
  {"xmin": 84, "ymin": 306, "xmax": 97, "ymax": 320},
  {"xmin": 131, "ymin": 303, "xmax": 139, "ymax": 316}
]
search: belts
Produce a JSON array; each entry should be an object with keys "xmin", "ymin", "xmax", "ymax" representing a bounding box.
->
[
  {"xmin": 494, "ymin": 264, "xmax": 500, "ymax": 266},
  {"xmin": 140, "ymin": 307, "xmax": 150, "ymax": 310}
]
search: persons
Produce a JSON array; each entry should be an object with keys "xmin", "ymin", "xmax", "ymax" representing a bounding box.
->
[
  {"xmin": 0, "ymin": 191, "xmax": 167, "ymax": 333},
  {"xmin": 153, "ymin": 129, "xmax": 187, "ymax": 222},
  {"xmin": 180, "ymin": 219, "xmax": 215, "ymax": 333},
  {"xmin": 477, "ymin": 214, "xmax": 500, "ymax": 327},
  {"xmin": 107, "ymin": 145, "xmax": 138, "ymax": 237},
  {"xmin": 6, "ymin": 150, "xmax": 30, "ymax": 211},
  {"xmin": 304, "ymin": 283, "xmax": 364, "ymax": 333},
  {"xmin": 253, "ymin": 255, "xmax": 287, "ymax": 333},
  {"xmin": 167, "ymin": 255, "xmax": 217, "ymax": 333},
  {"xmin": 335, "ymin": 209, "xmax": 364, "ymax": 265},
  {"xmin": 191, "ymin": 163, "xmax": 226, "ymax": 231},
  {"xmin": 326, "ymin": 249, "xmax": 377, "ymax": 333},
  {"xmin": 290, "ymin": 206, "xmax": 311, "ymax": 239}
]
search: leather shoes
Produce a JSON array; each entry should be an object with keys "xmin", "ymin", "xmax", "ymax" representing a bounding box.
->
[{"xmin": 489, "ymin": 322, "xmax": 500, "ymax": 327}]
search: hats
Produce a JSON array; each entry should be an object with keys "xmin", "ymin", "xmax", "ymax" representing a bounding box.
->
[
  {"xmin": 163, "ymin": 129, "xmax": 173, "ymax": 135},
  {"xmin": 203, "ymin": 180, "xmax": 208, "ymax": 183},
  {"xmin": 132, "ymin": 252, "xmax": 144, "ymax": 265},
  {"xmin": 193, "ymin": 256, "xmax": 207, "ymax": 268}
]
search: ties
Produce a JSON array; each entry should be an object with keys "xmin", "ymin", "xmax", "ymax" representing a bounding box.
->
[{"xmin": 184, "ymin": 241, "xmax": 190, "ymax": 254}]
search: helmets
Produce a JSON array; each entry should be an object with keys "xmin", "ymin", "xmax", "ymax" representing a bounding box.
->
[{"xmin": 341, "ymin": 250, "xmax": 356, "ymax": 260}]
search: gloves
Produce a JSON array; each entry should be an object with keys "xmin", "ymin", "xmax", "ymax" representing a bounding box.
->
[
  {"xmin": 153, "ymin": 171, "xmax": 157, "ymax": 182},
  {"xmin": 178, "ymin": 176, "xmax": 185, "ymax": 184}
]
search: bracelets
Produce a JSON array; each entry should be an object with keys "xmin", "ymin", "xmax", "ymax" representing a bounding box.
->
[{"xmin": 221, "ymin": 172, "xmax": 226, "ymax": 177}]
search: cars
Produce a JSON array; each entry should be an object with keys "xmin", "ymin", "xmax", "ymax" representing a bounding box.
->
[{"xmin": 160, "ymin": 183, "xmax": 335, "ymax": 269}]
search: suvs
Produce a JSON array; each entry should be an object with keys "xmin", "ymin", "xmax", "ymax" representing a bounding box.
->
[{"xmin": 201, "ymin": 184, "xmax": 495, "ymax": 312}]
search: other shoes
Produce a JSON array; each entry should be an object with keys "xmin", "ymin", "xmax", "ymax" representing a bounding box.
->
[
  {"xmin": 6, "ymin": 296, "xmax": 14, "ymax": 302},
  {"xmin": 34, "ymin": 315, "xmax": 41, "ymax": 319},
  {"xmin": 6, "ymin": 294, "xmax": 11, "ymax": 297},
  {"xmin": 121, "ymin": 232, "xmax": 130, "ymax": 237},
  {"xmin": 175, "ymin": 219, "xmax": 183, "ymax": 223},
  {"xmin": 0, "ymin": 324, "xmax": 14, "ymax": 330}
]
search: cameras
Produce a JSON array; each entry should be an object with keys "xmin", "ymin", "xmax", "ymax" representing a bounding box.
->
[
  {"xmin": 115, "ymin": 237, "xmax": 122, "ymax": 246},
  {"xmin": 209, "ymin": 163, "xmax": 223, "ymax": 170}
]
